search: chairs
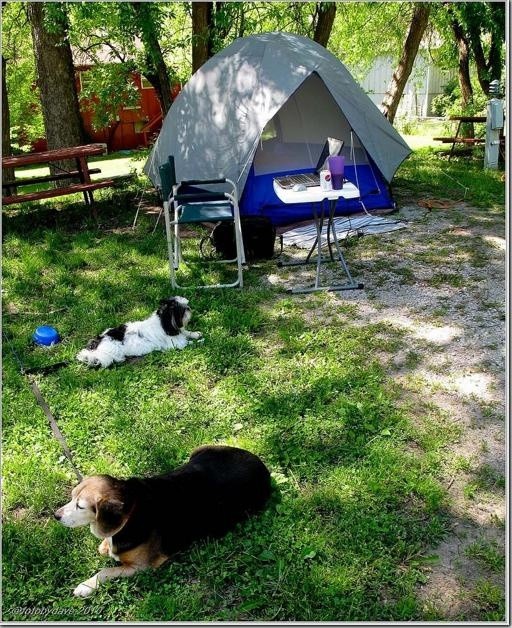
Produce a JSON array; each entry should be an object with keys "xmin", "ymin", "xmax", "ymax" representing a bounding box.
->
[{"xmin": 159, "ymin": 155, "xmax": 246, "ymax": 291}]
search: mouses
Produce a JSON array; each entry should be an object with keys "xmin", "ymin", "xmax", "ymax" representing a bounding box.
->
[{"xmin": 292, "ymin": 183, "xmax": 307, "ymax": 192}]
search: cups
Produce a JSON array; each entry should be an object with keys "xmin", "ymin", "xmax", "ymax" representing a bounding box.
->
[{"xmin": 327, "ymin": 155, "xmax": 346, "ymax": 191}]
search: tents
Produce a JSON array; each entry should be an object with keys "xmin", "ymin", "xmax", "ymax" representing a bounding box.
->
[{"xmin": 141, "ymin": 31, "xmax": 415, "ymax": 239}]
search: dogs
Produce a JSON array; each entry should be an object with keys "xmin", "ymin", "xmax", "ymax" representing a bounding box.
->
[
  {"xmin": 76, "ymin": 296, "xmax": 203, "ymax": 368},
  {"xmin": 54, "ymin": 446, "xmax": 271, "ymax": 596}
]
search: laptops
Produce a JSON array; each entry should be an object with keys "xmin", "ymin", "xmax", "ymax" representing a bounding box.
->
[{"xmin": 272, "ymin": 135, "xmax": 345, "ymax": 190}]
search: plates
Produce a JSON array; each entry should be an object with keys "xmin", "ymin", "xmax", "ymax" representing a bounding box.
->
[{"xmin": 32, "ymin": 326, "xmax": 60, "ymax": 346}]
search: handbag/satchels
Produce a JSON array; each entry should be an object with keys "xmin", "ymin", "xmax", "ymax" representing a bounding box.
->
[{"xmin": 200, "ymin": 216, "xmax": 283, "ymax": 264}]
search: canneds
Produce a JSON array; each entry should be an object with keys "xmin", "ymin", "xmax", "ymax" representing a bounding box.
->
[{"xmin": 320, "ymin": 170, "xmax": 332, "ymax": 191}]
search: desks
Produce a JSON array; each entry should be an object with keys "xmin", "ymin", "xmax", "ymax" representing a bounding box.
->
[
  {"xmin": 449, "ymin": 116, "xmax": 505, "ymax": 164},
  {"xmin": 2, "ymin": 144, "xmax": 106, "ymax": 227},
  {"xmin": 273, "ymin": 174, "xmax": 364, "ymax": 294}
]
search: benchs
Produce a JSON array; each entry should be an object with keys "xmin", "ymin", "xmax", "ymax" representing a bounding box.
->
[
  {"xmin": 1, "ymin": 178, "xmax": 115, "ymax": 225},
  {"xmin": 433, "ymin": 136, "xmax": 506, "ymax": 164},
  {"xmin": 3, "ymin": 168, "xmax": 102, "ymax": 205}
]
